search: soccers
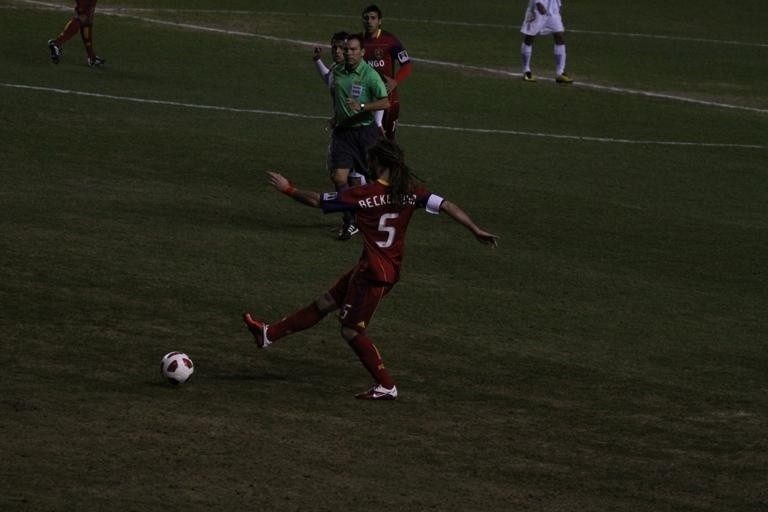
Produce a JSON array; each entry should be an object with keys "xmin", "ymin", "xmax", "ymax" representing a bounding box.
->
[{"xmin": 161, "ymin": 351, "xmax": 194, "ymax": 385}]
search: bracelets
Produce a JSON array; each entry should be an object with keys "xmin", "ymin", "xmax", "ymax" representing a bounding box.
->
[{"xmin": 284, "ymin": 187, "xmax": 293, "ymax": 195}]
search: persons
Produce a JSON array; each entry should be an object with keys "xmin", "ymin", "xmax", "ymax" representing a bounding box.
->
[
  {"xmin": 312, "ymin": 4, "xmax": 411, "ymax": 241},
  {"xmin": 240, "ymin": 140, "xmax": 500, "ymax": 401},
  {"xmin": 46, "ymin": 0, "xmax": 106, "ymax": 69},
  {"xmin": 521, "ymin": 1, "xmax": 575, "ymax": 85}
]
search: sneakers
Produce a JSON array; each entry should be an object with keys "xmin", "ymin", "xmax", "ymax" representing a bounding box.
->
[
  {"xmin": 88, "ymin": 56, "xmax": 106, "ymax": 67},
  {"xmin": 525, "ymin": 72, "xmax": 536, "ymax": 81},
  {"xmin": 555, "ymin": 73, "xmax": 573, "ymax": 82},
  {"xmin": 354, "ymin": 384, "xmax": 398, "ymax": 401},
  {"xmin": 244, "ymin": 313, "xmax": 273, "ymax": 349},
  {"xmin": 49, "ymin": 40, "xmax": 62, "ymax": 66},
  {"xmin": 338, "ymin": 223, "xmax": 359, "ymax": 238}
]
